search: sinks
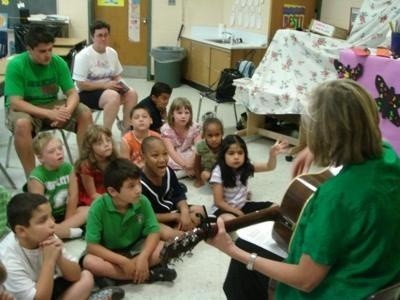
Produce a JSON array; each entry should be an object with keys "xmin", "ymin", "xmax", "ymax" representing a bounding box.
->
[{"xmin": 208, "ymin": 39, "xmax": 235, "ymax": 43}]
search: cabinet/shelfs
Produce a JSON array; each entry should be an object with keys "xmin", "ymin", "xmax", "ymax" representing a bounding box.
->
[{"xmin": 179, "ymin": 35, "xmax": 267, "ymax": 91}]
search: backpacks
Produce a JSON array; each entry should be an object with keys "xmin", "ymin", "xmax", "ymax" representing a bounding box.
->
[{"xmin": 216, "ymin": 59, "xmax": 256, "ymax": 102}]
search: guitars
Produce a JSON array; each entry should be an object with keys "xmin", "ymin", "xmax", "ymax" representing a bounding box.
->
[{"xmin": 158, "ymin": 165, "xmax": 343, "ymax": 266}]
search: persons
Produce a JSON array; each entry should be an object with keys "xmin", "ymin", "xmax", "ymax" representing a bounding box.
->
[
  {"xmin": 120, "ymin": 105, "xmax": 162, "ymax": 170},
  {"xmin": 28, "ymin": 132, "xmax": 90, "ymax": 239},
  {"xmin": 193, "ymin": 109, "xmax": 227, "ymax": 188},
  {"xmin": 72, "ymin": 21, "xmax": 138, "ymax": 134},
  {"xmin": 130, "ymin": 82, "xmax": 172, "ymax": 134},
  {"xmin": 79, "ymin": 158, "xmax": 177, "ymax": 289},
  {"xmin": 140, "ymin": 136, "xmax": 205, "ymax": 242},
  {"xmin": 205, "ymin": 79, "xmax": 400, "ymax": 299},
  {"xmin": 159, "ymin": 97, "xmax": 202, "ymax": 179},
  {"xmin": 4, "ymin": 28, "xmax": 93, "ymax": 192},
  {"xmin": 210, "ymin": 135, "xmax": 290, "ymax": 223},
  {"xmin": 0, "ymin": 193, "xmax": 125, "ymax": 300},
  {"xmin": 74, "ymin": 124, "xmax": 119, "ymax": 206}
]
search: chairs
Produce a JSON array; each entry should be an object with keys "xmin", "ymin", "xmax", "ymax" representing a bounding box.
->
[
  {"xmin": 196, "ymin": 60, "xmax": 256, "ymax": 123},
  {"xmin": 71, "ymin": 45, "xmax": 120, "ymax": 123},
  {"xmin": 1, "ymin": 96, "xmax": 75, "ymax": 166}
]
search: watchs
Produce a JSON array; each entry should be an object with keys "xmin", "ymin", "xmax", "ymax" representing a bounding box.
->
[{"xmin": 246, "ymin": 253, "xmax": 258, "ymax": 270}]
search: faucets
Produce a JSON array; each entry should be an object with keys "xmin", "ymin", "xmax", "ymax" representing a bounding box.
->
[{"xmin": 221, "ymin": 31, "xmax": 234, "ymax": 40}]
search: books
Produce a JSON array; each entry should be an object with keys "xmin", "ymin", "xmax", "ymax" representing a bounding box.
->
[{"xmin": 118, "ymin": 81, "xmax": 130, "ymax": 95}]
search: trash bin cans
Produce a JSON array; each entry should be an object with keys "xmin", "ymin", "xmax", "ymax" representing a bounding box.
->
[{"xmin": 152, "ymin": 45, "xmax": 185, "ymax": 89}]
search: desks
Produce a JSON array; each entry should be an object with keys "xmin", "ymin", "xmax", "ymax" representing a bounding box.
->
[
  {"xmin": 28, "ymin": 14, "xmax": 69, "ymax": 38},
  {"xmin": 53, "ymin": 36, "xmax": 86, "ymax": 50},
  {"xmin": 0, "ymin": 56, "xmax": 11, "ymax": 107},
  {"xmin": 52, "ymin": 48, "xmax": 72, "ymax": 64}
]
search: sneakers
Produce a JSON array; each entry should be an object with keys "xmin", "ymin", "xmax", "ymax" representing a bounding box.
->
[
  {"xmin": 147, "ymin": 265, "xmax": 176, "ymax": 283},
  {"xmin": 89, "ymin": 285, "xmax": 125, "ymax": 300}
]
